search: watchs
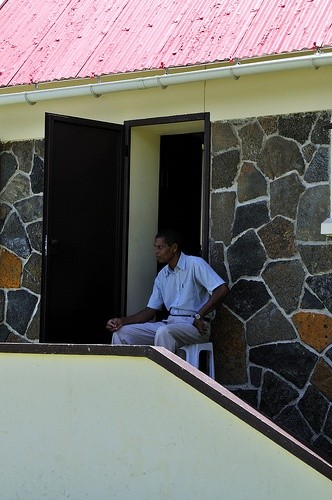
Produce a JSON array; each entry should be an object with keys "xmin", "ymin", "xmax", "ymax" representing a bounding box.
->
[{"xmin": 194, "ymin": 313, "xmax": 202, "ymax": 320}]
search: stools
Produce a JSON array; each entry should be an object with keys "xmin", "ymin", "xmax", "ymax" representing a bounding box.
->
[{"xmin": 179, "ymin": 343, "xmax": 214, "ymax": 380}]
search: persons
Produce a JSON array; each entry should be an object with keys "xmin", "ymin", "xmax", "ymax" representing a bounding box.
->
[{"xmin": 106, "ymin": 230, "xmax": 228, "ymax": 355}]
